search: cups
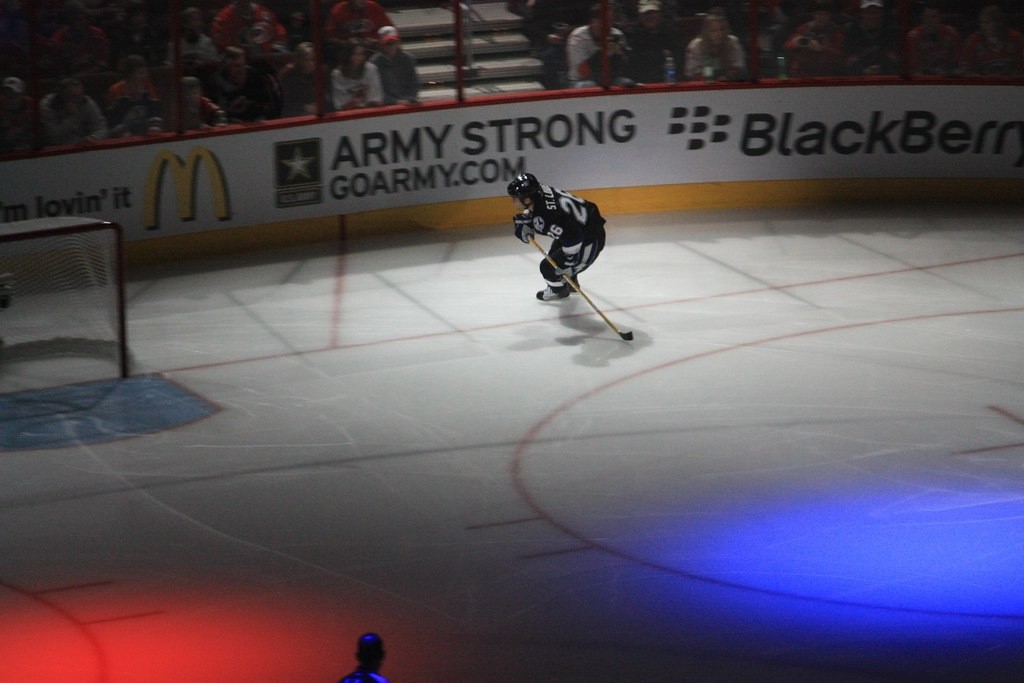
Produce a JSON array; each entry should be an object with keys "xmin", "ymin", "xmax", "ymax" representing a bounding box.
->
[{"xmin": 552, "ymin": 23, "xmax": 569, "ymax": 42}]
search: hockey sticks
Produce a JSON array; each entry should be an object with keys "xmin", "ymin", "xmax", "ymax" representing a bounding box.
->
[{"xmin": 528, "ymin": 234, "xmax": 635, "ymax": 341}]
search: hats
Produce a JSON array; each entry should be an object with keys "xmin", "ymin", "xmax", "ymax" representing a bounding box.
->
[
  {"xmin": 860, "ymin": 0, "xmax": 883, "ymax": 9},
  {"xmin": 378, "ymin": 25, "xmax": 400, "ymax": 44},
  {"xmin": 2, "ymin": 78, "xmax": 25, "ymax": 93},
  {"xmin": 637, "ymin": 0, "xmax": 661, "ymax": 13}
]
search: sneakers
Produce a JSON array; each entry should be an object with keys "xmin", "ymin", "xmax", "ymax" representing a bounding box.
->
[
  {"xmin": 536, "ymin": 285, "xmax": 571, "ymax": 302},
  {"xmin": 569, "ymin": 276, "xmax": 580, "ymax": 295}
]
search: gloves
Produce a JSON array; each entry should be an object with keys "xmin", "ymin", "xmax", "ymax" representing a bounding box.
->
[
  {"xmin": 513, "ymin": 213, "xmax": 535, "ymax": 244},
  {"xmin": 555, "ymin": 257, "xmax": 576, "ymax": 283}
]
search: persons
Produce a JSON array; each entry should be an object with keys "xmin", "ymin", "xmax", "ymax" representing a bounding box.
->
[
  {"xmin": 329, "ymin": 38, "xmax": 386, "ymax": 112},
  {"xmin": 369, "ymin": 24, "xmax": 420, "ymax": 106},
  {"xmin": 506, "ymin": 172, "xmax": 609, "ymax": 302},
  {"xmin": 336, "ymin": 631, "xmax": 390, "ymax": 683},
  {"xmin": 682, "ymin": 14, "xmax": 749, "ymax": 81},
  {"xmin": 0, "ymin": 0, "xmax": 1024, "ymax": 143}
]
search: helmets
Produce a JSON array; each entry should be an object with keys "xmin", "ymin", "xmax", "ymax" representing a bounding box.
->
[{"xmin": 508, "ymin": 173, "xmax": 541, "ymax": 202}]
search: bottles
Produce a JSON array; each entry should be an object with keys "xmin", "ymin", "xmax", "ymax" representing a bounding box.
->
[
  {"xmin": 663, "ymin": 57, "xmax": 676, "ymax": 82},
  {"xmin": 775, "ymin": 57, "xmax": 788, "ymax": 80},
  {"xmin": 702, "ymin": 50, "xmax": 714, "ymax": 81}
]
